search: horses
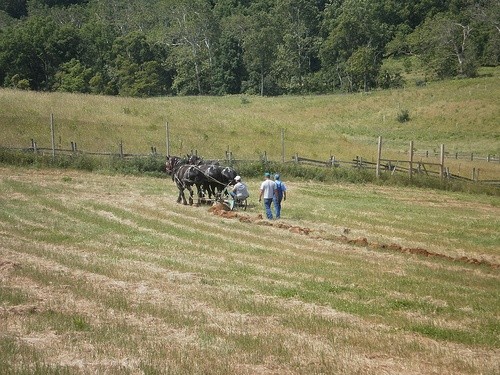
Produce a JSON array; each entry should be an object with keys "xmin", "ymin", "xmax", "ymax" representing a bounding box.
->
[{"xmin": 165, "ymin": 153, "xmax": 237, "ymax": 207}]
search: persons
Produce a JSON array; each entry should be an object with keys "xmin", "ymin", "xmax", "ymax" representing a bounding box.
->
[
  {"xmin": 229, "ymin": 176, "xmax": 249, "ymax": 208},
  {"xmin": 272, "ymin": 174, "xmax": 287, "ymax": 219},
  {"xmin": 258, "ymin": 172, "xmax": 278, "ymax": 221}
]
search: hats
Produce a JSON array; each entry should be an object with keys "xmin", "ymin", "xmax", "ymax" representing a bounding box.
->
[
  {"xmin": 234, "ymin": 176, "xmax": 241, "ymax": 180},
  {"xmin": 274, "ymin": 175, "xmax": 279, "ymax": 179},
  {"xmin": 264, "ymin": 173, "xmax": 270, "ymax": 177}
]
